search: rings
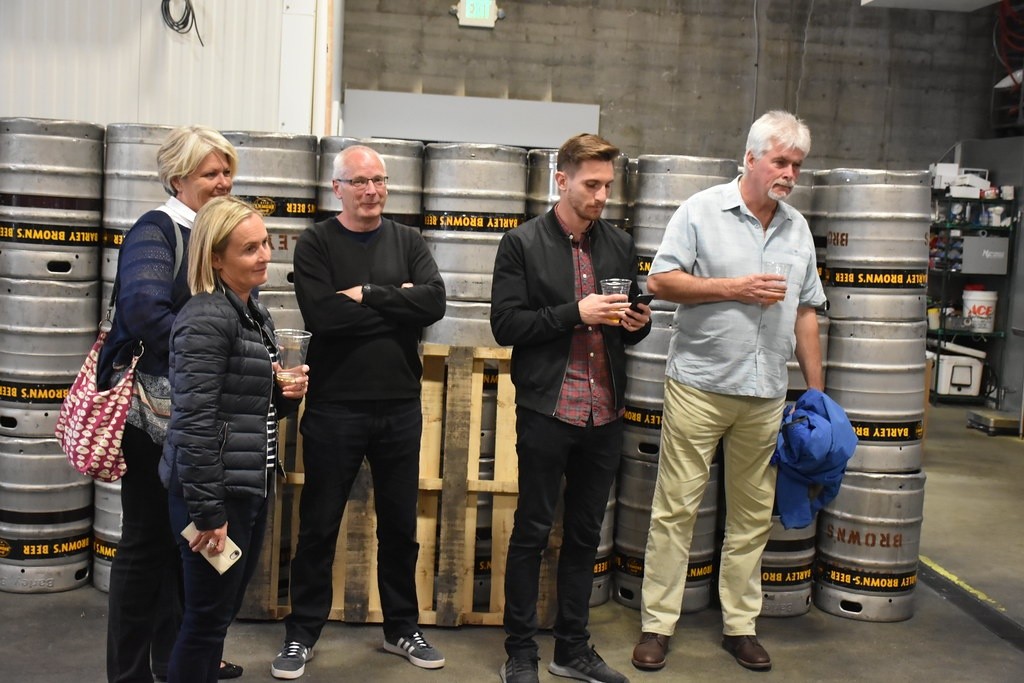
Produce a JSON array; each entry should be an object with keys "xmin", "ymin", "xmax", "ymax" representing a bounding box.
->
[
  {"xmin": 207, "ymin": 541, "xmax": 217, "ymax": 550},
  {"xmin": 301, "ymin": 383, "xmax": 304, "ymax": 388}
]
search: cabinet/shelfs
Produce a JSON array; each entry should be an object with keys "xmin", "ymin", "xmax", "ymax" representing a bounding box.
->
[{"xmin": 925, "ymin": 185, "xmax": 1020, "ymax": 408}]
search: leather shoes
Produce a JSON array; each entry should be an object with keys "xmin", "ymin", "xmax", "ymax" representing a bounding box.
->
[
  {"xmin": 632, "ymin": 633, "xmax": 669, "ymax": 669},
  {"xmin": 722, "ymin": 632, "xmax": 773, "ymax": 671}
]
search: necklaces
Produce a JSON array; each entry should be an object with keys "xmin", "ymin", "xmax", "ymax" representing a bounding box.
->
[{"xmin": 763, "ymin": 228, "xmax": 767, "ymax": 230}]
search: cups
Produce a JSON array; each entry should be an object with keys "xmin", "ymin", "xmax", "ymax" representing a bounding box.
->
[
  {"xmin": 272, "ymin": 327, "xmax": 313, "ymax": 382},
  {"xmin": 764, "ymin": 261, "xmax": 793, "ymax": 301},
  {"xmin": 599, "ymin": 277, "xmax": 633, "ymax": 325}
]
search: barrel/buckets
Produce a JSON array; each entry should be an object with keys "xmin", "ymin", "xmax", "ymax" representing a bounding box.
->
[
  {"xmin": 961, "ymin": 290, "xmax": 997, "ymax": 333},
  {"xmin": 0, "ymin": 118, "xmax": 930, "ymax": 627}
]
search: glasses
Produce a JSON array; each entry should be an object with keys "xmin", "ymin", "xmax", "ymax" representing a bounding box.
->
[{"xmin": 337, "ymin": 177, "xmax": 388, "ymax": 189}]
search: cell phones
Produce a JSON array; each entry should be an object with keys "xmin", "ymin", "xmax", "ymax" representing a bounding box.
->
[
  {"xmin": 180, "ymin": 521, "xmax": 241, "ymax": 576},
  {"xmin": 619, "ymin": 294, "xmax": 655, "ymax": 324}
]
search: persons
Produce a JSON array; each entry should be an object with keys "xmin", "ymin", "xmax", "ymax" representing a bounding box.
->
[
  {"xmin": 490, "ymin": 133, "xmax": 652, "ymax": 683},
  {"xmin": 632, "ymin": 112, "xmax": 826, "ymax": 683},
  {"xmin": 272, "ymin": 146, "xmax": 446, "ymax": 678},
  {"xmin": 157, "ymin": 196, "xmax": 309, "ymax": 683},
  {"xmin": 96, "ymin": 124, "xmax": 243, "ymax": 683}
]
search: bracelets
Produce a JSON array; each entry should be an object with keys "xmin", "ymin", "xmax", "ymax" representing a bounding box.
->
[{"xmin": 361, "ymin": 283, "xmax": 370, "ymax": 306}]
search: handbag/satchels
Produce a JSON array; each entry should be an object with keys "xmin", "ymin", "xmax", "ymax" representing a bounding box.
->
[{"xmin": 55, "ymin": 323, "xmax": 145, "ymax": 484}]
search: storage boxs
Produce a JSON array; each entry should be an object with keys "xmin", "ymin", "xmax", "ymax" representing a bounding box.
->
[
  {"xmin": 926, "ymin": 338, "xmax": 987, "ymax": 396},
  {"xmin": 928, "ymin": 162, "xmax": 1015, "ymax": 200},
  {"xmin": 952, "ymin": 230, "xmax": 1009, "ymax": 273}
]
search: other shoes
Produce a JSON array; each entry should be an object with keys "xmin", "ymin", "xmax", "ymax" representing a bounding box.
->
[{"xmin": 218, "ymin": 660, "xmax": 244, "ymax": 679}]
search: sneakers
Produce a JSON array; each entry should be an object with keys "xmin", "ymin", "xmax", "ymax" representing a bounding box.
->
[
  {"xmin": 270, "ymin": 640, "xmax": 314, "ymax": 679},
  {"xmin": 498, "ymin": 656, "xmax": 539, "ymax": 683},
  {"xmin": 383, "ymin": 632, "xmax": 446, "ymax": 669},
  {"xmin": 550, "ymin": 645, "xmax": 630, "ymax": 683}
]
